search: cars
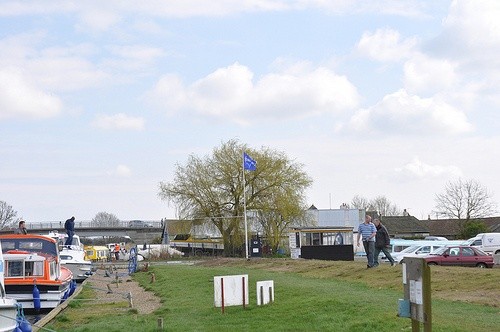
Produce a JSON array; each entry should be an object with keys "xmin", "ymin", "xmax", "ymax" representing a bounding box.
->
[
  {"xmin": 422, "ymin": 245, "xmax": 495, "ymax": 268},
  {"xmin": 384, "ymin": 241, "xmax": 459, "ymax": 264}
]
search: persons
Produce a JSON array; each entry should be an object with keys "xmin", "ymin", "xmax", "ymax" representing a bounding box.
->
[
  {"xmin": 373, "ymin": 219, "xmax": 396, "ymax": 267},
  {"xmin": 357, "ymin": 214, "xmax": 377, "ymax": 268},
  {"xmin": 14, "ymin": 221, "xmax": 25, "ymax": 234},
  {"xmin": 64, "ymin": 217, "xmax": 75, "ymax": 250},
  {"xmin": 114, "ymin": 244, "xmax": 120, "ymax": 260}
]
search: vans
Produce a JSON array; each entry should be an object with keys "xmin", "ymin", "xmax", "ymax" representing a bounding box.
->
[{"xmin": 381, "ymin": 239, "xmax": 415, "ymax": 260}]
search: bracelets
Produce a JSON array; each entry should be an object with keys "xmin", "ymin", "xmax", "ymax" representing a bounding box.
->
[{"xmin": 369, "ymin": 236, "xmax": 372, "ymax": 239}]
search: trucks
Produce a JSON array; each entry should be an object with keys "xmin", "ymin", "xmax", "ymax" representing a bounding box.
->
[
  {"xmin": 424, "ymin": 236, "xmax": 448, "ymax": 242},
  {"xmin": 461, "ymin": 232, "xmax": 500, "ymax": 254}
]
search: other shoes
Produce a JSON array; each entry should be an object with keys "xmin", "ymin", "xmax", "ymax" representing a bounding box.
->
[
  {"xmin": 367, "ymin": 264, "xmax": 373, "ymax": 268},
  {"xmin": 391, "ymin": 262, "xmax": 395, "ymax": 266},
  {"xmin": 374, "ymin": 263, "xmax": 379, "ymax": 267}
]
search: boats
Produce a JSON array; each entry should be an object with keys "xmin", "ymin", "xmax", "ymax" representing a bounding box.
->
[
  {"xmin": 0, "ymin": 243, "xmax": 21, "ymax": 332},
  {"xmin": 48, "ymin": 230, "xmax": 92, "ymax": 282},
  {"xmin": 0, "ymin": 234, "xmax": 76, "ymax": 309}
]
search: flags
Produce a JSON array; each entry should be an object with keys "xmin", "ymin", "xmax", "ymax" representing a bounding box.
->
[{"xmin": 243, "ymin": 151, "xmax": 257, "ymax": 171}]
